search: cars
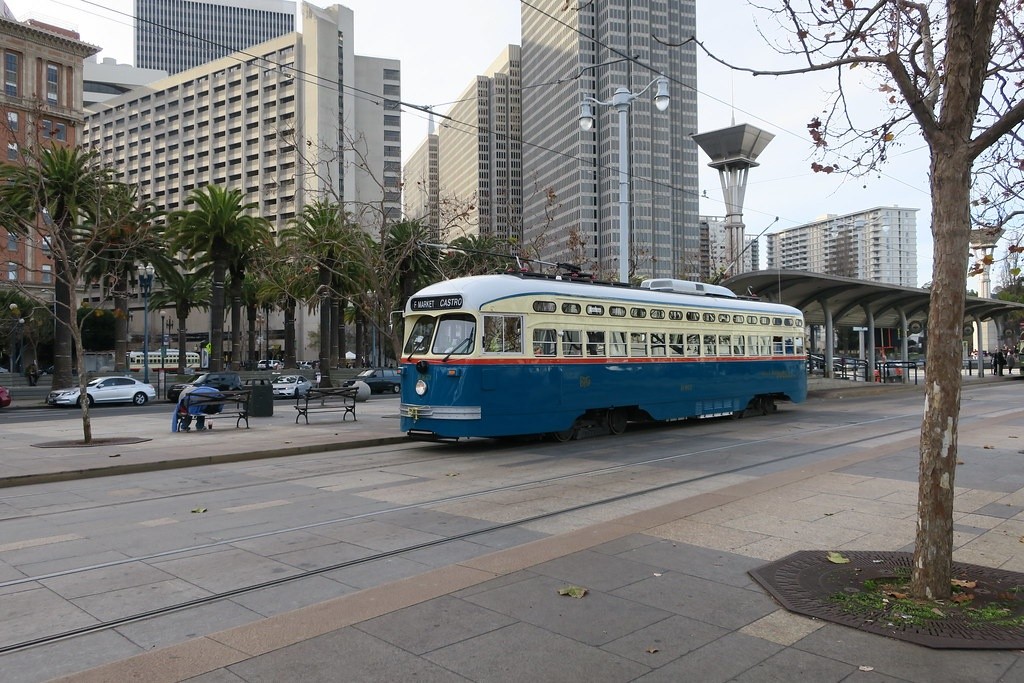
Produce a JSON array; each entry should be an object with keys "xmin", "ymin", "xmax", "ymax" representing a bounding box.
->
[
  {"xmin": 343, "ymin": 368, "xmax": 402, "ymax": 394},
  {"xmin": 0, "ymin": 367, "xmax": 8, "ymax": 373},
  {"xmin": 48, "ymin": 365, "xmax": 77, "ymax": 374},
  {"xmin": 0, "ymin": 386, "xmax": 11, "ymax": 409},
  {"xmin": 806, "ymin": 353, "xmax": 925, "ymax": 377},
  {"xmin": 257, "ymin": 359, "xmax": 321, "ymax": 369},
  {"xmin": 272, "ymin": 375, "xmax": 313, "ymax": 399},
  {"xmin": 46, "ymin": 377, "xmax": 157, "ymax": 409}
]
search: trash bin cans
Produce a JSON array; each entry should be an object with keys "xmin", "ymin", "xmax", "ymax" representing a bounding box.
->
[{"xmin": 242, "ymin": 378, "xmax": 273, "ymax": 417}]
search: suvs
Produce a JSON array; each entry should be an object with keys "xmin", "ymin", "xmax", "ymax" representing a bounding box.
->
[{"xmin": 167, "ymin": 371, "xmax": 243, "ymax": 403}]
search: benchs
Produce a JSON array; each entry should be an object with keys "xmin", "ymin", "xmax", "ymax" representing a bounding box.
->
[
  {"xmin": 176, "ymin": 390, "xmax": 253, "ymax": 433},
  {"xmin": 293, "ymin": 385, "xmax": 359, "ymax": 424}
]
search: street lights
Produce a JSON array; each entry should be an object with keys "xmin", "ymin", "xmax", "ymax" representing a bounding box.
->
[
  {"xmin": 578, "ymin": 70, "xmax": 673, "ymax": 285},
  {"xmin": 10, "ymin": 302, "xmax": 25, "ymax": 377},
  {"xmin": 158, "ymin": 310, "xmax": 168, "ymax": 353},
  {"xmin": 137, "ymin": 262, "xmax": 156, "ymax": 384},
  {"xmin": 41, "ymin": 288, "xmax": 57, "ymax": 340}
]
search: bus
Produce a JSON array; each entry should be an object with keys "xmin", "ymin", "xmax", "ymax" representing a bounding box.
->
[
  {"xmin": 99, "ymin": 350, "xmax": 200, "ymax": 372},
  {"xmin": 397, "ymin": 273, "xmax": 810, "ymax": 448},
  {"xmin": 1016, "ymin": 331, "xmax": 1024, "ymax": 381},
  {"xmin": 163, "ymin": 335, "xmax": 173, "ymax": 345}
]
